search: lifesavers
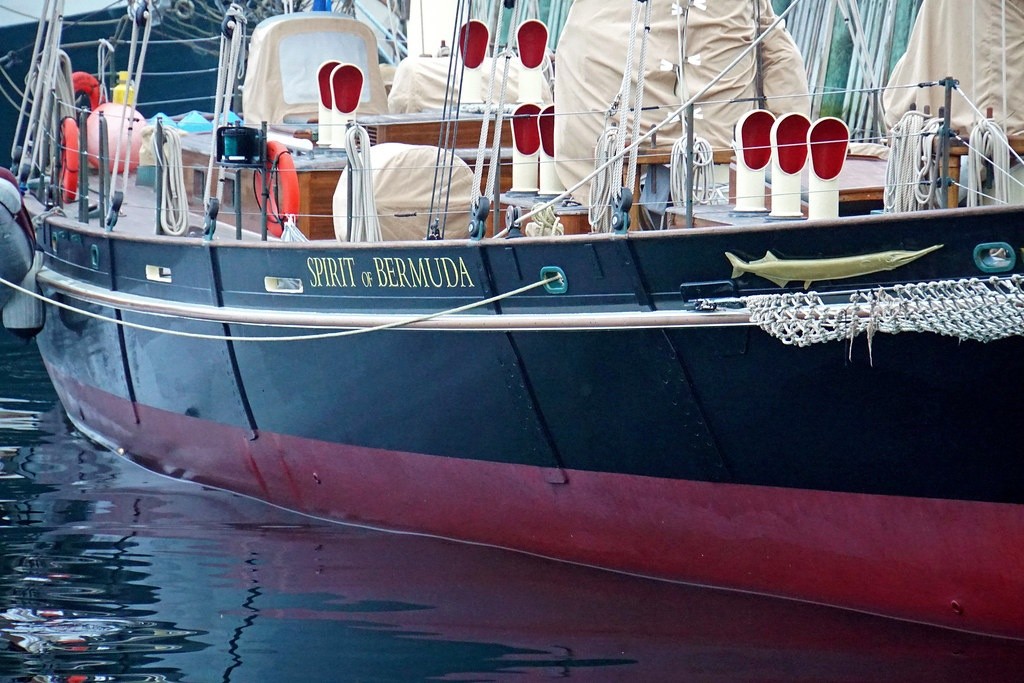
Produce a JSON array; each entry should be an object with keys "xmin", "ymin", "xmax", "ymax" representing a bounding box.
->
[
  {"xmin": 255, "ymin": 139, "xmax": 300, "ymax": 238},
  {"xmin": 58, "ymin": 116, "xmax": 80, "ymax": 204},
  {"xmin": 71, "ymin": 71, "xmax": 109, "ymax": 136}
]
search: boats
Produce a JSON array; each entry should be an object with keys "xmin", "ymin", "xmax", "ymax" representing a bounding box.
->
[{"xmin": 0, "ymin": 0, "xmax": 1024, "ymax": 654}]
